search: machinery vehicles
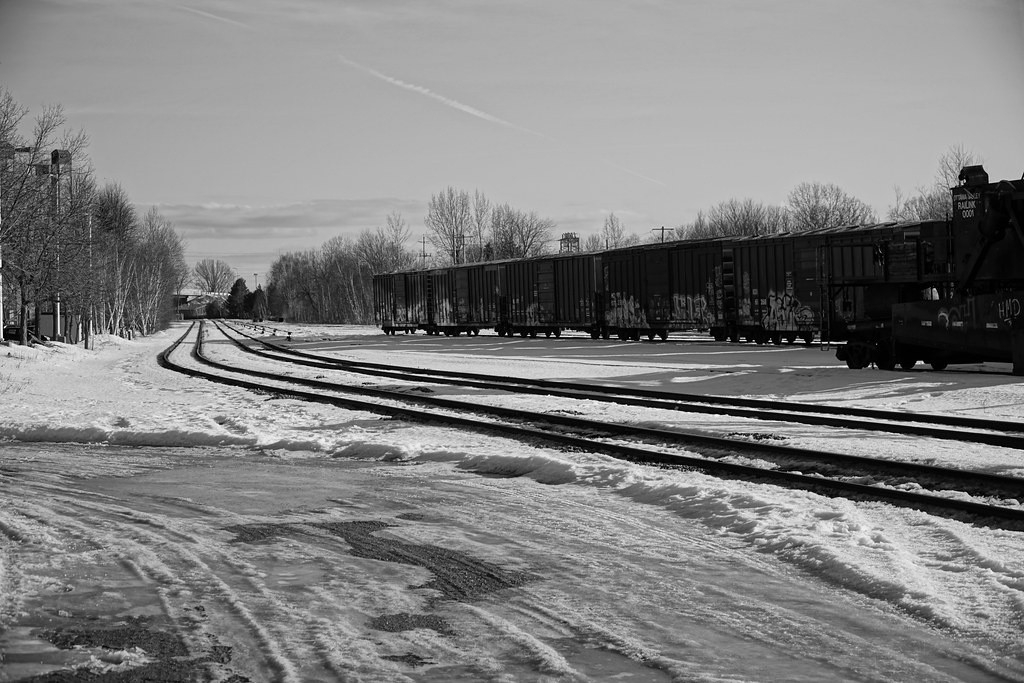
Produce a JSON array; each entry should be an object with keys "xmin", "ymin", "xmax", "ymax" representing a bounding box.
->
[{"xmin": 835, "ymin": 190, "xmax": 1023, "ymax": 377}]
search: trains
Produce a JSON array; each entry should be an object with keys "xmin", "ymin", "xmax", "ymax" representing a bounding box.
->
[{"xmin": 372, "ymin": 164, "xmax": 1023, "ymax": 345}]
